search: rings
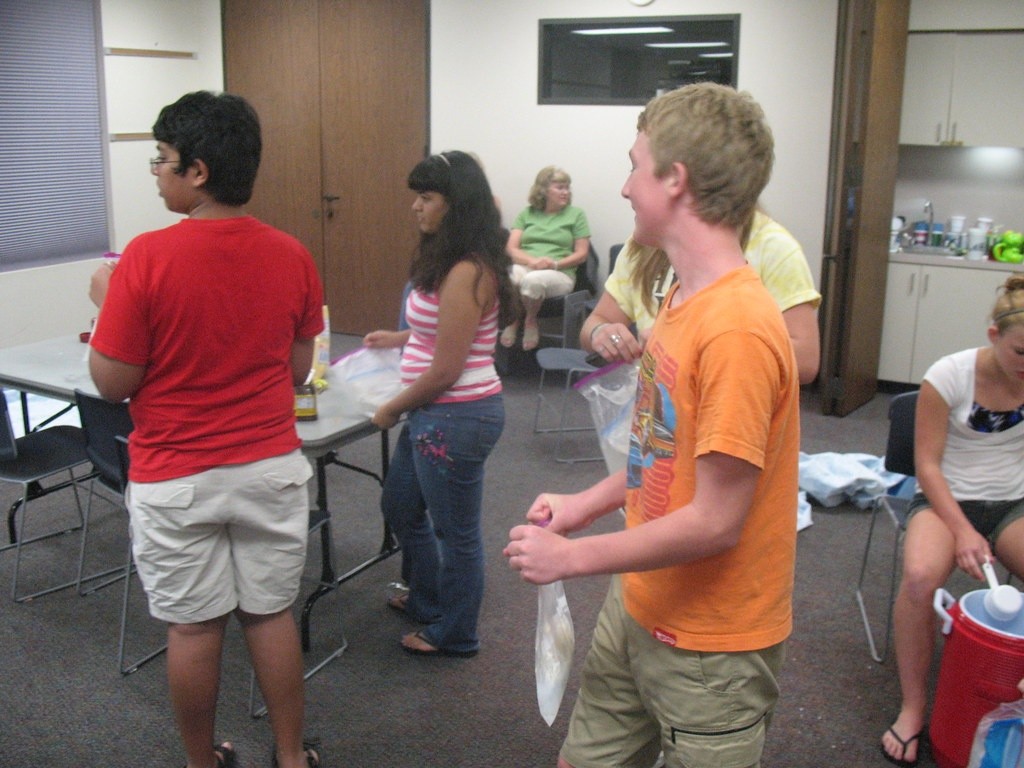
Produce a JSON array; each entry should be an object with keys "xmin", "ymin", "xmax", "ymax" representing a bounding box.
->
[{"xmin": 610, "ymin": 334, "xmax": 621, "ymax": 344}]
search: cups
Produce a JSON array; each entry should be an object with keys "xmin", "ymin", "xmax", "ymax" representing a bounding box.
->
[
  {"xmin": 950, "ymin": 216, "xmax": 966, "ymax": 233},
  {"xmin": 976, "ymin": 217, "xmax": 994, "ymax": 231}
]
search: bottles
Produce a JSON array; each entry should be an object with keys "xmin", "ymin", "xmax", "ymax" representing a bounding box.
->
[
  {"xmin": 931, "ymin": 223, "xmax": 943, "ymax": 245},
  {"xmin": 914, "ymin": 222, "xmax": 929, "ymax": 247},
  {"xmin": 294, "ymin": 384, "xmax": 317, "ymax": 421},
  {"xmin": 966, "ymin": 228, "xmax": 987, "ymax": 255}
]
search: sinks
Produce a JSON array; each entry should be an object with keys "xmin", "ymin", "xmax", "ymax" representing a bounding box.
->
[{"xmin": 891, "ymin": 247, "xmax": 967, "ymax": 260}]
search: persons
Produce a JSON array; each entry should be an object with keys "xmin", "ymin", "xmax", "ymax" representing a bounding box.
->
[
  {"xmin": 879, "ymin": 276, "xmax": 1024, "ymax": 766},
  {"xmin": 502, "ymin": 168, "xmax": 589, "ymax": 350},
  {"xmin": 577, "ymin": 205, "xmax": 819, "ymax": 388},
  {"xmin": 504, "ymin": 82, "xmax": 800, "ymax": 768},
  {"xmin": 89, "ymin": 90, "xmax": 324, "ymax": 768},
  {"xmin": 357, "ymin": 151, "xmax": 517, "ymax": 660}
]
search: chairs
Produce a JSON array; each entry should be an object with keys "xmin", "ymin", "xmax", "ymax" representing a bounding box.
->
[
  {"xmin": 497, "ymin": 239, "xmax": 599, "ymax": 381},
  {"xmin": 0, "ymin": 389, "xmax": 139, "ymax": 602},
  {"xmin": 534, "ymin": 305, "xmax": 638, "ymax": 465},
  {"xmin": 73, "ymin": 393, "xmax": 349, "ymax": 720},
  {"xmin": 854, "ymin": 390, "xmax": 921, "ymax": 664}
]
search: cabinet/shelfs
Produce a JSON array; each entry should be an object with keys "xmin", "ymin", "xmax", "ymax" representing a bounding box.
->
[
  {"xmin": 898, "ymin": 32, "xmax": 1022, "ymax": 149},
  {"xmin": 875, "ymin": 261, "xmax": 1019, "ymax": 384}
]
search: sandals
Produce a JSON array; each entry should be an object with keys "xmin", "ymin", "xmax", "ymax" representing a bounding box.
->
[
  {"xmin": 271, "ymin": 746, "xmax": 323, "ymax": 768},
  {"xmin": 183, "ymin": 745, "xmax": 236, "ymax": 768}
]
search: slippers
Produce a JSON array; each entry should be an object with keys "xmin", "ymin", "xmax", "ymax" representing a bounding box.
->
[
  {"xmin": 391, "ymin": 594, "xmax": 444, "ymax": 622},
  {"xmin": 401, "ymin": 631, "xmax": 479, "ymax": 657},
  {"xmin": 521, "ymin": 321, "xmax": 540, "ymax": 351},
  {"xmin": 500, "ymin": 321, "xmax": 521, "ymax": 348},
  {"xmin": 879, "ymin": 718, "xmax": 928, "ymax": 768}
]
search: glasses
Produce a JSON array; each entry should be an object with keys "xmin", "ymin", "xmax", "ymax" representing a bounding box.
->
[{"xmin": 149, "ymin": 155, "xmax": 189, "ymax": 176}]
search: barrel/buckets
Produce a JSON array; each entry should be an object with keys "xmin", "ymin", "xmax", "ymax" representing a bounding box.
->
[{"xmin": 928, "ymin": 586, "xmax": 1024, "ymax": 768}]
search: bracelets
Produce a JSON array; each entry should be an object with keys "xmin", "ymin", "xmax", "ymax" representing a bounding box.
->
[
  {"xmin": 554, "ymin": 261, "xmax": 560, "ymax": 271},
  {"xmin": 590, "ymin": 322, "xmax": 607, "ymax": 341}
]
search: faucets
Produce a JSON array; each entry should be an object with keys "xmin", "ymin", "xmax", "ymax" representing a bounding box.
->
[{"xmin": 924, "ymin": 201, "xmax": 935, "ymax": 247}]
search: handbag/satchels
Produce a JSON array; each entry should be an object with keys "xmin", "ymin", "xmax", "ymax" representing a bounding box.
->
[
  {"xmin": 535, "ymin": 576, "xmax": 576, "ymax": 723},
  {"xmin": 574, "ymin": 354, "xmax": 645, "ymax": 476}
]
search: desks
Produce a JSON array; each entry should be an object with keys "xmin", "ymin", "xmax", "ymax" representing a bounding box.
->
[{"xmin": 0, "ymin": 325, "xmax": 417, "ymax": 653}]
